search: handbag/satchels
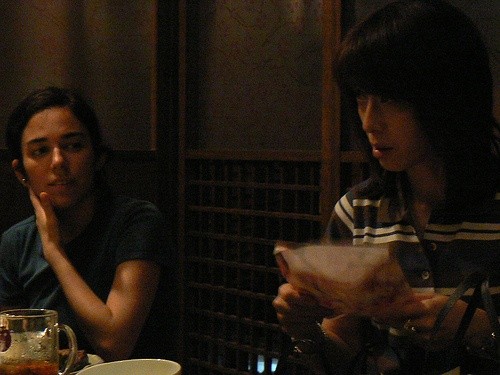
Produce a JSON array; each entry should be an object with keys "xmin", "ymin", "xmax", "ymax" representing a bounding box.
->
[{"xmin": 460, "ymin": 280, "xmax": 500, "ymax": 375}]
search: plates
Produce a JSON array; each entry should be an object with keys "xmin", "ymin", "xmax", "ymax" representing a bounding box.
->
[{"xmin": 59, "ymin": 353, "xmax": 104, "ymax": 375}]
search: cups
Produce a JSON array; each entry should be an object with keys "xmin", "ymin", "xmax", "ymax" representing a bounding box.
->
[{"xmin": 0, "ymin": 309, "xmax": 77, "ymax": 375}]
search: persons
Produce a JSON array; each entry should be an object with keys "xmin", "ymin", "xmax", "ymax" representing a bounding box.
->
[
  {"xmin": 0, "ymin": 86, "xmax": 179, "ymax": 362},
  {"xmin": 271, "ymin": 0, "xmax": 500, "ymax": 375}
]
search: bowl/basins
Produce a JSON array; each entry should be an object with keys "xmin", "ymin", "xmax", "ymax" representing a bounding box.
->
[{"xmin": 75, "ymin": 359, "xmax": 182, "ymax": 375}]
